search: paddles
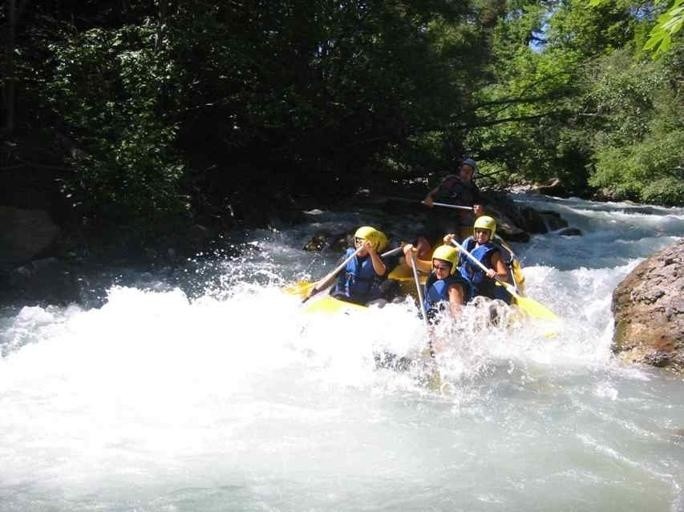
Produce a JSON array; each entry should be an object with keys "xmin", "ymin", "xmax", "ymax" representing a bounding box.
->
[
  {"xmin": 283, "ymin": 247, "xmax": 402, "ymax": 299},
  {"xmin": 449, "ymin": 239, "xmax": 560, "ymax": 339}
]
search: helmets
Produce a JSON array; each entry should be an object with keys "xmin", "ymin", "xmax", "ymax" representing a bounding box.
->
[
  {"xmin": 474, "ymin": 216, "xmax": 496, "ymax": 240},
  {"xmin": 464, "ymin": 158, "xmax": 476, "ymax": 169},
  {"xmin": 431, "ymin": 245, "xmax": 457, "ymax": 275},
  {"xmin": 354, "ymin": 226, "xmax": 387, "ymax": 253}
]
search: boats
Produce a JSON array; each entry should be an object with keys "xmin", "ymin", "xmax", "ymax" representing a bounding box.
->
[{"xmin": 305, "ymin": 227, "xmax": 525, "ymax": 350}]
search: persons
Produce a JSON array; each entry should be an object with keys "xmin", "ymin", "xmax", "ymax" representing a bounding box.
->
[{"xmin": 306, "ymin": 158, "xmax": 511, "ymax": 328}]
